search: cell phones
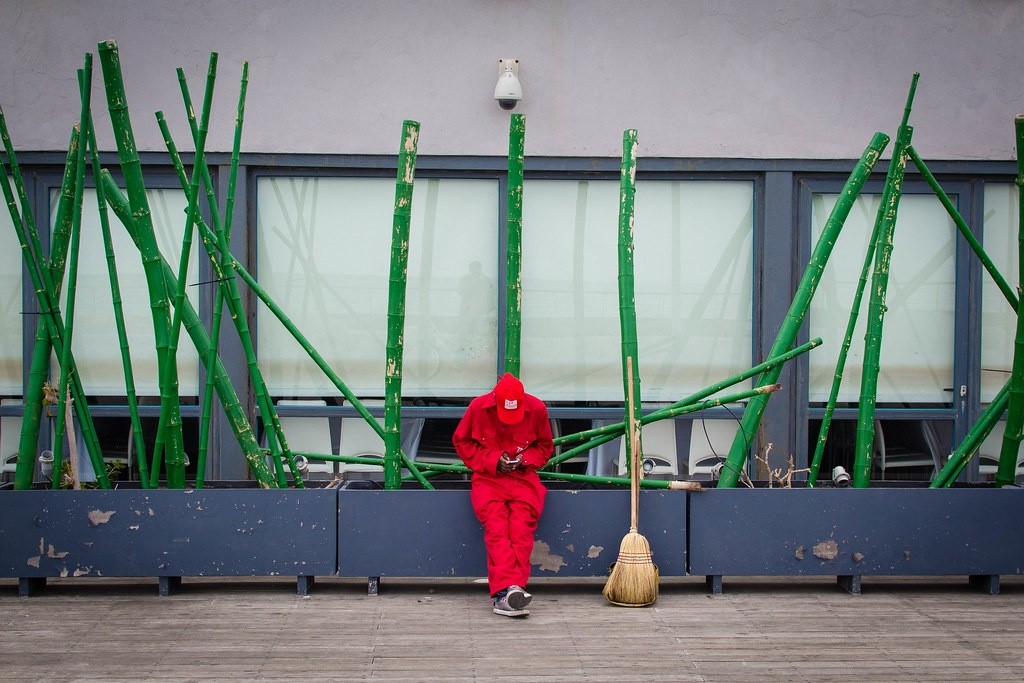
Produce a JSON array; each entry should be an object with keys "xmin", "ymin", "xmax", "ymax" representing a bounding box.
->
[{"xmin": 508, "ymin": 460, "xmax": 518, "ymax": 465}]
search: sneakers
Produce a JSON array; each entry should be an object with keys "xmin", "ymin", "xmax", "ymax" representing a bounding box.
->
[
  {"xmin": 493, "ymin": 598, "xmax": 530, "ymax": 616},
  {"xmin": 505, "ymin": 583, "xmax": 532, "ymax": 609}
]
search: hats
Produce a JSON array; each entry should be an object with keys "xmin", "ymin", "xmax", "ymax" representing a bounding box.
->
[{"xmin": 496, "ymin": 374, "xmax": 524, "ymax": 424}]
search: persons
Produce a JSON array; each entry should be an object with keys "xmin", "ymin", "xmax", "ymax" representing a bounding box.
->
[{"xmin": 451, "ymin": 372, "xmax": 555, "ymax": 616}]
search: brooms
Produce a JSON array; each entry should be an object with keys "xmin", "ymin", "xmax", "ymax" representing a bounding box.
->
[{"xmin": 603, "ymin": 356, "xmax": 658, "ymax": 604}]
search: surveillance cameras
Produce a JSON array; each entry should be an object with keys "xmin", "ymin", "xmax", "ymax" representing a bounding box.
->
[{"xmin": 494, "ymin": 72, "xmax": 523, "ymax": 110}]
